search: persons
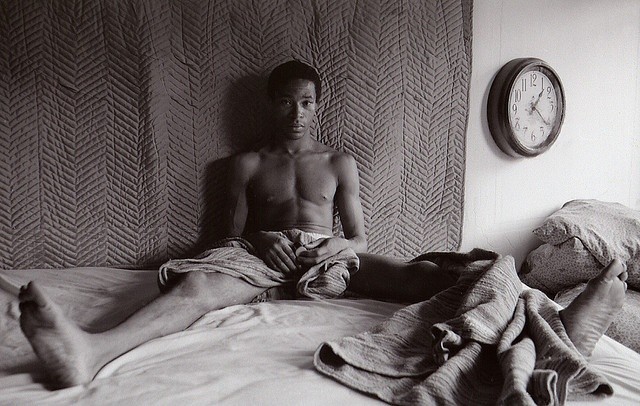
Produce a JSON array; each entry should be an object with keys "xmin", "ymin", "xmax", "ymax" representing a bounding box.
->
[{"xmin": 18, "ymin": 59, "xmax": 629, "ymax": 389}]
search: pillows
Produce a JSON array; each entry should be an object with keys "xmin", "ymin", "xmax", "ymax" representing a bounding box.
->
[
  {"xmin": 519, "ymin": 238, "xmax": 639, "ymax": 290},
  {"xmin": 535, "ymin": 196, "xmax": 640, "ymax": 272},
  {"xmin": 555, "ymin": 282, "xmax": 639, "ymax": 349}
]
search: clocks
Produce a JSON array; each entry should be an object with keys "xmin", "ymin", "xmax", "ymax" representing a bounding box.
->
[{"xmin": 494, "ymin": 60, "xmax": 568, "ymax": 160}]
